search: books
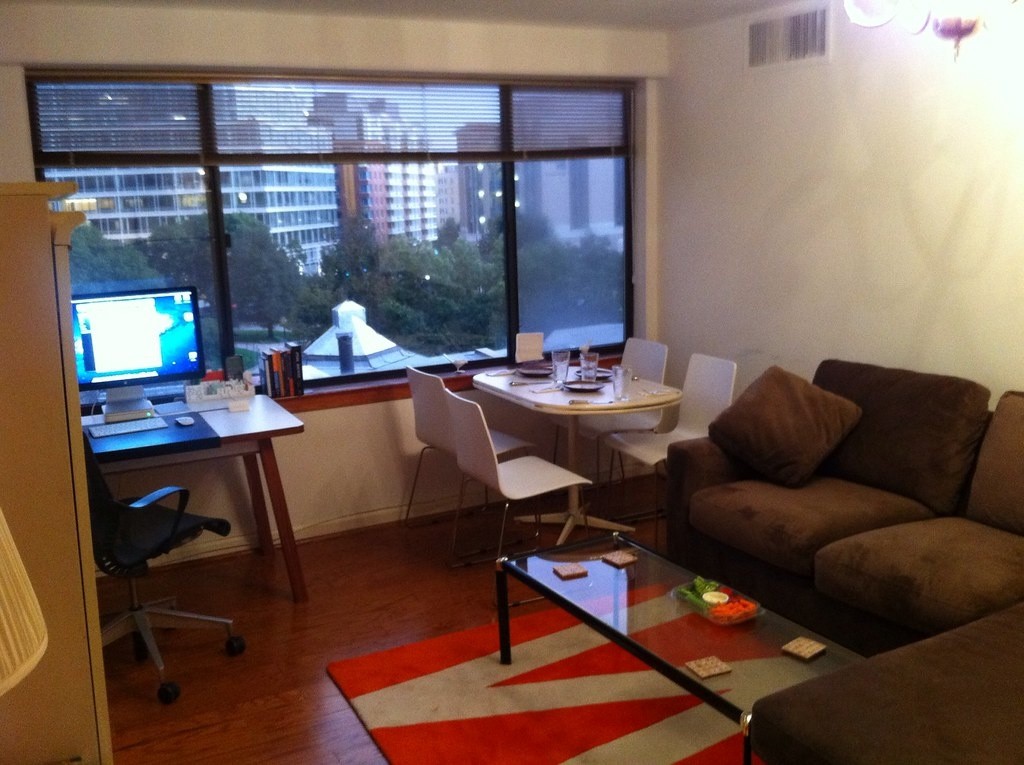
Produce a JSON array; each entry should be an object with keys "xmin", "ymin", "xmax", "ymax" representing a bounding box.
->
[{"xmin": 258, "ymin": 341, "xmax": 304, "ymax": 399}]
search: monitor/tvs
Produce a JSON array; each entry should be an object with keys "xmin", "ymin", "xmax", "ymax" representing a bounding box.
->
[{"xmin": 70, "ymin": 285, "xmax": 206, "ymax": 402}]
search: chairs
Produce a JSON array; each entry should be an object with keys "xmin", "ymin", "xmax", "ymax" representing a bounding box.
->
[
  {"xmin": 405, "ymin": 365, "xmax": 540, "ymax": 559},
  {"xmin": 444, "ymin": 388, "xmax": 593, "ymax": 608},
  {"xmin": 81, "ymin": 428, "xmax": 246, "ymax": 705},
  {"xmin": 605, "ymin": 353, "xmax": 737, "ymax": 545},
  {"xmin": 553, "ymin": 337, "xmax": 669, "ymax": 510}
]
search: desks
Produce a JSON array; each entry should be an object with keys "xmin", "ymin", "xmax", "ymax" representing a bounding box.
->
[
  {"xmin": 81, "ymin": 394, "xmax": 308, "ymax": 604},
  {"xmin": 494, "ymin": 535, "xmax": 866, "ymax": 765},
  {"xmin": 472, "ymin": 366, "xmax": 683, "ymax": 545}
]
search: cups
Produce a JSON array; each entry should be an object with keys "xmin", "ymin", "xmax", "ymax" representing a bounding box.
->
[
  {"xmin": 612, "ymin": 364, "xmax": 632, "ymax": 402},
  {"xmin": 552, "ymin": 350, "xmax": 570, "ymax": 391},
  {"xmin": 580, "ymin": 353, "xmax": 599, "ymax": 382}
]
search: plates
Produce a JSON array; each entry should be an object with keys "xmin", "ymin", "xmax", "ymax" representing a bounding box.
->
[
  {"xmin": 563, "ymin": 381, "xmax": 605, "ymax": 393},
  {"xmin": 576, "ymin": 368, "xmax": 613, "ymax": 380},
  {"xmin": 675, "ymin": 577, "xmax": 765, "ymax": 623},
  {"xmin": 517, "ymin": 366, "xmax": 554, "ymax": 377}
]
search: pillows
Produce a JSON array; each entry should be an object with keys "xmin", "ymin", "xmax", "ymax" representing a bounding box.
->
[{"xmin": 708, "ymin": 364, "xmax": 862, "ymax": 487}]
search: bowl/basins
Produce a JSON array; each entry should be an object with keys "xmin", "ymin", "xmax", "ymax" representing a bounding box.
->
[{"xmin": 702, "ymin": 592, "xmax": 729, "ymax": 606}]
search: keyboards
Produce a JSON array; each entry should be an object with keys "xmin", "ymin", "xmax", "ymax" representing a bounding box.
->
[{"xmin": 88, "ymin": 418, "xmax": 169, "ymax": 438}]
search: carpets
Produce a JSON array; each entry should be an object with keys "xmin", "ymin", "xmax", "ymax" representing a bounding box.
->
[{"xmin": 326, "ymin": 584, "xmax": 867, "ymax": 765}]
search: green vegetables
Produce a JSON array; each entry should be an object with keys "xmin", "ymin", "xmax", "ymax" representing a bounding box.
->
[{"xmin": 677, "ymin": 576, "xmax": 719, "ymax": 610}]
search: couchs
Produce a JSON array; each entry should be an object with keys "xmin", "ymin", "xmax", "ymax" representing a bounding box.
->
[{"xmin": 668, "ymin": 359, "xmax": 1024, "ymax": 765}]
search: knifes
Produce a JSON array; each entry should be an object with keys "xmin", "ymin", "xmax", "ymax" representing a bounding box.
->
[
  {"xmin": 568, "ymin": 399, "xmax": 614, "ymax": 404},
  {"xmin": 510, "ymin": 381, "xmax": 551, "ymax": 385}
]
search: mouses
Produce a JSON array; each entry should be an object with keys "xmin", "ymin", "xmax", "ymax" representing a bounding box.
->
[{"xmin": 174, "ymin": 416, "xmax": 195, "ymax": 426}]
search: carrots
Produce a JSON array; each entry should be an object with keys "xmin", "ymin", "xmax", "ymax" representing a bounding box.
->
[{"xmin": 708, "ymin": 599, "xmax": 756, "ymax": 617}]
